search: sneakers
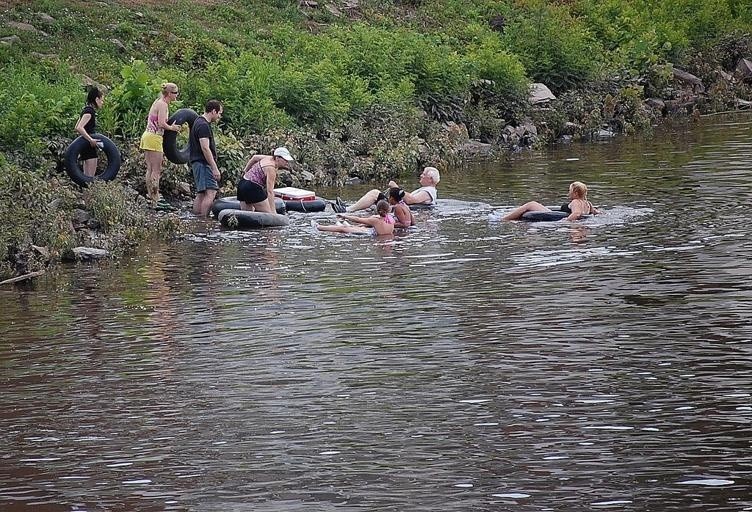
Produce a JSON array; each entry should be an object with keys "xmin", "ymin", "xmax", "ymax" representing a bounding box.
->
[{"xmin": 332, "ymin": 196, "xmax": 347, "ymax": 214}]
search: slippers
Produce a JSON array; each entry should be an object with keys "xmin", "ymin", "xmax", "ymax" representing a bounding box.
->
[{"xmin": 311, "ymin": 219, "xmax": 319, "ymax": 232}]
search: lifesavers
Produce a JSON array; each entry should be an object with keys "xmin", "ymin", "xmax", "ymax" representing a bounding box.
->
[
  {"xmin": 163, "ymin": 109, "xmax": 199, "ymax": 164},
  {"xmin": 283, "ymin": 199, "xmax": 326, "ymax": 212},
  {"xmin": 212, "ymin": 196, "xmax": 288, "ymax": 227},
  {"xmin": 64, "ymin": 133, "xmax": 120, "ymax": 188},
  {"xmin": 409, "ymin": 203, "xmax": 434, "ymax": 210},
  {"xmin": 520, "ymin": 205, "xmax": 594, "ymax": 221}
]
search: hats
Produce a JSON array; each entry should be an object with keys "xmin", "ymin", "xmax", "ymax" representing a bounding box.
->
[{"xmin": 274, "ymin": 146, "xmax": 294, "ymax": 161}]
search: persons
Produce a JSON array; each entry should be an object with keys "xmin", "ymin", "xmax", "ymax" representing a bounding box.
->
[
  {"xmin": 502, "ymin": 181, "xmax": 600, "ymax": 224},
  {"xmin": 74, "ymin": 87, "xmax": 104, "ymax": 177},
  {"xmin": 190, "ymin": 99, "xmax": 224, "ymax": 215},
  {"xmin": 140, "ymin": 82, "xmax": 181, "ymax": 207},
  {"xmin": 238, "ymin": 147, "xmax": 292, "ymax": 214},
  {"xmin": 310, "ymin": 167, "xmax": 441, "ymax": 236}
]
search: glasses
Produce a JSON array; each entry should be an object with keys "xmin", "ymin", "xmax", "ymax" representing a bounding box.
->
[{"xmin": 172, "ymin": 92, "xmax": 179, "ymax": 94}]
